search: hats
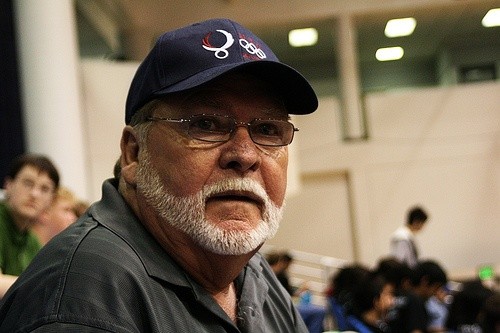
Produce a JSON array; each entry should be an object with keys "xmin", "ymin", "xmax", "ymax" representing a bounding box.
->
[{"xmin": 126, "ymin": 18, "xmax": 318, "ymax": 125}]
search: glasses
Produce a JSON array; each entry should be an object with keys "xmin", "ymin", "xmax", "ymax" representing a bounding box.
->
[{"xmin": 145, "ymin": 114, "xmax": 299, "ymax": 146}]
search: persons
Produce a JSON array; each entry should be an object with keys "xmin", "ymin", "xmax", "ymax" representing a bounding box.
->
[
  {"xmin": 389, "ymin": 207, "xmax": 427, "ymax": 267},
  {"xmin": 296, "ymin": 257, "xmax": 500, "ymax": 333},
  {"xmin": 0, "ymin": 18, "xmax": 318, "ymax": 333},
  {"xmin": 266, "ymin": 252, "xmax": 309, "ymax": 296},
  {"xmin": 0, "ymin": 153, "xmax": 60, "ymax": 300}
]
